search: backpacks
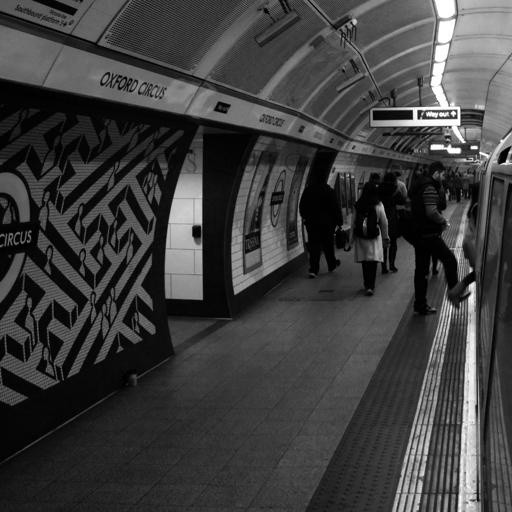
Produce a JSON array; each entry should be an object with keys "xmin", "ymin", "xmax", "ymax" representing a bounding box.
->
[{"xmin": 355, "ymin": 199, "xmax": 379, "ymax": 240}]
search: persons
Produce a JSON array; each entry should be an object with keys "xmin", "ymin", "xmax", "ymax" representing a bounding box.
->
[{"xmin": 297, "ymin": 158, "xmax": 484, "ymax": 314}]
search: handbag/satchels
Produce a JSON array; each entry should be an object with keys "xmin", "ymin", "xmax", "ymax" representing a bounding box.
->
[
  {"xmin": 335, "ymin": 227, "xmax": 351, "ymax": 252},
  {"xmin": 397, "ymin": 210, "xmax": 414, "ymax": 243}
]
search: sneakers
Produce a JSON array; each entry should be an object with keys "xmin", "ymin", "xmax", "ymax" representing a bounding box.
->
[
  {"xmin": 366, "ymin": 289, "xmax": 373, "ymax": 296},
  {"xmin": 328, "ymin": 260, "xmax": 340, "ymax": 270},
  {"xmin": 310, "ymin": 270, "xmax": 319, "ymax": 278}
]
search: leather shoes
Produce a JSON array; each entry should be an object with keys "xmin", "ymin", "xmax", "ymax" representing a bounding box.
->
[
  {"xmin": 454, "ymin": 291, "xmax": 470, "ymax": 308},
  {"xmin": 417, "ymin": 304, "xmax": 437, "ymax": 314}
]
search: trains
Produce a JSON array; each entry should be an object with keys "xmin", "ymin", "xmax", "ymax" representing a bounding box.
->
[{"xmin": 467, "ymin": 125, "xmax": 512, "ymax": 512}]
